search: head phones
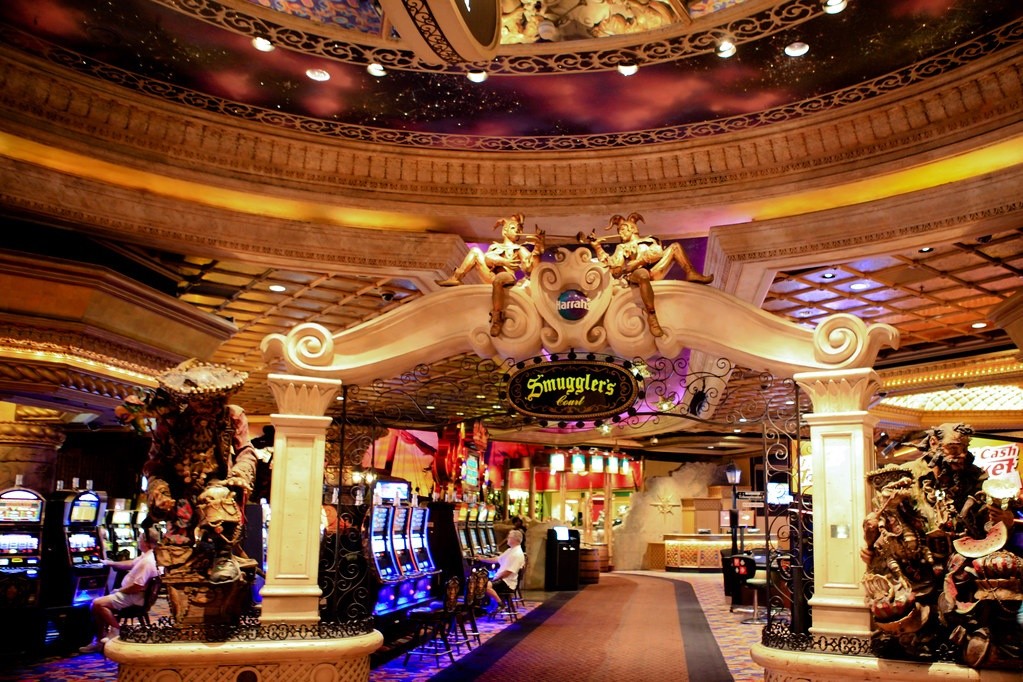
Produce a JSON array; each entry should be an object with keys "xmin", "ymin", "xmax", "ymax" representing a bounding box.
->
[{"xmin": 143, "ymin": 528, "xmax": 150, "ymax": 542}]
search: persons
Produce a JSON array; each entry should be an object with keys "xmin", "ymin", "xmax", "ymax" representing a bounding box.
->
[
  {"xmin": 79, "ymin": 526, "xmax": 158, "ymax": 653},
  {"xmin": 434, "ymin": 212, "xmax": 546, "ymax": 337},
  {"xmin": 511, "ymin": 516, "xmax": 528, "ymax": 592},
  {"xmin": 571, "ymin": 512, "xmax": 583, "ymax": 527},
  {"xmin": 586, "ymin": 212, "xmax": 714, "ymax": 336},
  {"xmin": 474, "ymin": 530, "xmax": 526, "ymax": 613},
  {"xmin": 140, "ymin": 360, "xmax": 256, "ymax": 579},
  {"xmin": 900, "ymin": 423, "xmax": 989, "ymax": 541}
]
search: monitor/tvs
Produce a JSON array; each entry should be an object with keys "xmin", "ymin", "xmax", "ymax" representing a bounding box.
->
[
  {"xmin": 767, "ymin": 483, "xmax": 794, "ymax": 505},
  {"xmin": 554, "ymin": 526, "xmax": 569, "ymax": 541},
  {"xmin": 374, "ymin": 481, "xmax": 412, "ymax": 503}
]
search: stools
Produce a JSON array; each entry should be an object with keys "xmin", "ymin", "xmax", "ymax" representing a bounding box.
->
[{"xmin": 740, "ymin": 577, "xmax": 770, "ymax": 628}]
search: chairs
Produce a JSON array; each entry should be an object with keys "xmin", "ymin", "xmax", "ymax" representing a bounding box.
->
[
  {"xmin": 113, "ymin": 574, "xmax": 162, "ymax": 629},
  {"xmin": 400, "ymin": 552, "xmax": 530, "ymax": 669}
]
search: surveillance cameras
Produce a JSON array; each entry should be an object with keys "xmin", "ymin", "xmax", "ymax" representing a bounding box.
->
[
  {"xmin": 760, "ymin": 383, "xmax": 770, "ymax": 390},
  {"xmin": 878, "ymin": 391, "xmax": 889, "ymax": 397},
  {"xmin": 975, "ymin": 235, "xmax": 992, "ymax": 243},
  {"xmin": 379, "ymin": 291, "xmax": 395, "ymax": 302},
  {"xmin": 954, "ymin": 382, "xmax": 965, "ymax": 388}
]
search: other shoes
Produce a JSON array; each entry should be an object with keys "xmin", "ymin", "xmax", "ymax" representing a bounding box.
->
[
  {"xmin": 80, "ymin": 642, "xmax": 104, "ymax": 653},
  {"xmin": 491, "ymin": 603, "xmax": 509, "ymax": 614},
  {"xmin": 100, "ymin": 628, "xmax": 119, "ymax": 642}
]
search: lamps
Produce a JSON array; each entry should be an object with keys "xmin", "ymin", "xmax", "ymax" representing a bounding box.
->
[{"xmin": 725, "ymin": 461, "xmax": 742, "ymax": 557}]
